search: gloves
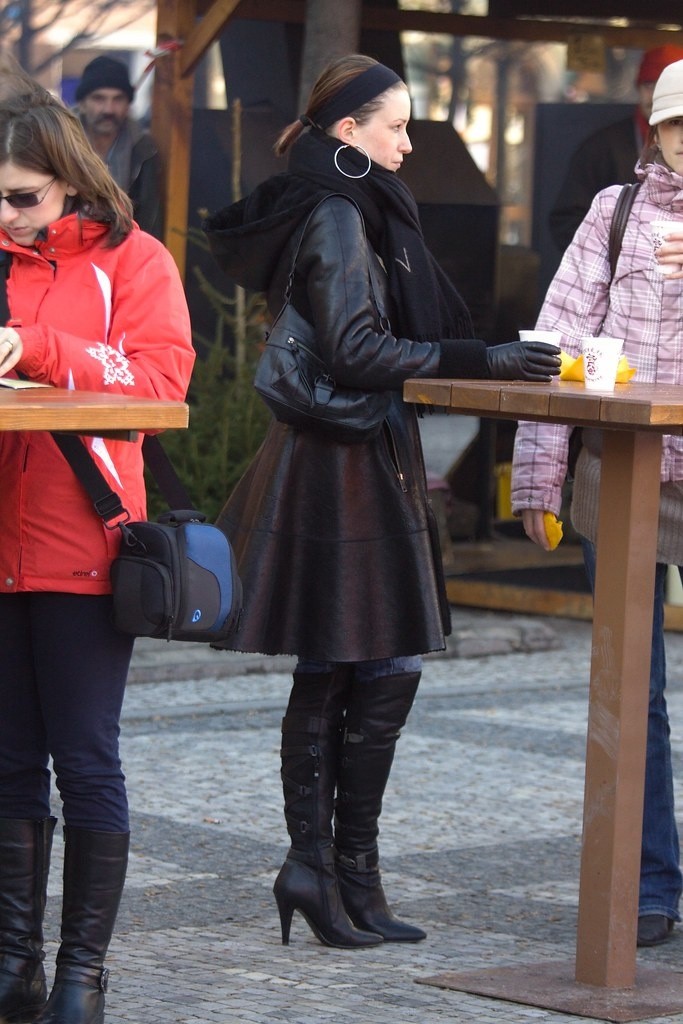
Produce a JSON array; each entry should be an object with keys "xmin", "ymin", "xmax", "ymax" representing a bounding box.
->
[{"xmin": 486, "ymin": 341, "xmax": 562, "ymax": 382}]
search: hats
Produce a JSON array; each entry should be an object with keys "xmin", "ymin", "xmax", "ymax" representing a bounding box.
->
[
  {"xmin": 648, "ymin": 60, "xmax": 683, "ymax": 126},
  {"xmin": 636, "ymin": 46, "xmax": 683, "ymax": 87},
  {"xmin": 77, "ymin": 56, "xmax": 135, "ymax": 102}
]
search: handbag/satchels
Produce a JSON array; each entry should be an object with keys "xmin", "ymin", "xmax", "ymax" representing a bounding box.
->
[
  {"xmin": 568, "ymin": 427, "xmax": 583, "ymax": 479},
  {"xmin": 255, "ymin": 193, "xmax": 393, "ymax": 441},
  {"xmin": 109, "ymin": 511, "xmax": 244, "ymax": 643}
]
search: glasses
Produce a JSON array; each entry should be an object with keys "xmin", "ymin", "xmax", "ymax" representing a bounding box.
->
[{"xmin": 0, "ymin": 176, "xmax": 57, "ymax": 208}]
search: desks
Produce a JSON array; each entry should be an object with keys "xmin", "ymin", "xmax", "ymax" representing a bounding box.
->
[
  {"xmin": 0, "ymin": 378, "xmax": 192, "ymax": 442},
  {"xmin": 402, "ymin": 377, "xmax": 683, "ymax": 1024}
]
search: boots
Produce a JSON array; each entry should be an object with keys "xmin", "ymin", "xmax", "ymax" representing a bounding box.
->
[
  {"xmin": 273, "ymin": 671, "xmax": 422, "ymax": 951},
  {"xmin": 0, "ymin": 817, "xmax": 130, "ymax": 1024}
]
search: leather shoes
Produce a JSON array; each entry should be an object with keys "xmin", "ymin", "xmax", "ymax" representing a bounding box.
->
[{"xmin": 637, "ymin": 914, "xmax": 673, "ymax": 945}]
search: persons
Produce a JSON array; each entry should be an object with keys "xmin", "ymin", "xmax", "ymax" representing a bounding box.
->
[
  {"xmin": 549, "ymin": 46, "xmax": 683, "ymax": 273},
  {"xmin": 70, "ymin": 55, "xmax": 164, "ymax": 246},
  {"xmin": 204, "ymin": 54, "xmax": 562, "ymax": 949},
  {"xmin": 510, "ymin": 59, "xmax": 683, "ymax": 947},
  {"xmin": 0, "ymin": 34, "xmax": 195, "ymax": 1024}
]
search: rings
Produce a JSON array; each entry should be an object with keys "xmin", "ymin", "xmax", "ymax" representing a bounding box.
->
[{"xmin": 6, "ymin": 340, "xmax": 14, "ymax": 349}]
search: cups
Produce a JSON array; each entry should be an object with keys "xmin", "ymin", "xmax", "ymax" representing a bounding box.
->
[
  {"xmin": 649, "ymin": 219, "xmax": 683, "ymax": 274},
  {"xmin": 580, "ymin": 337, "xmax": 624, "ymax": 391},
  {"xmin": 518, "ymin": 331, "xmax": 561, "ymax": 378}
]
